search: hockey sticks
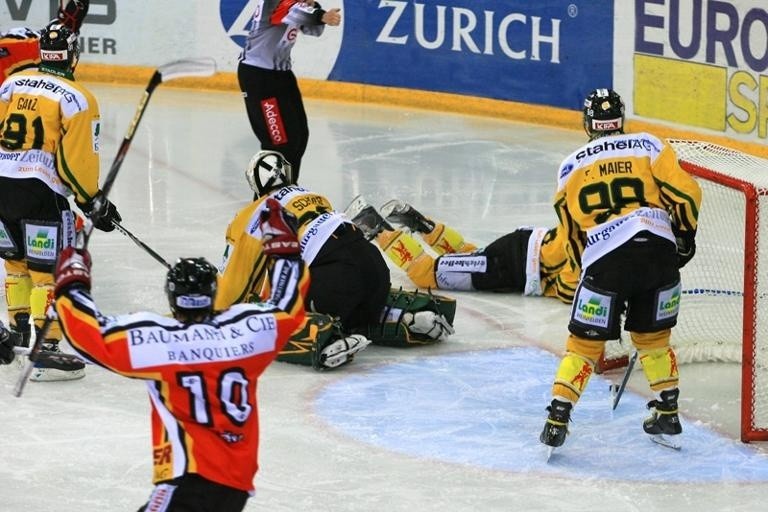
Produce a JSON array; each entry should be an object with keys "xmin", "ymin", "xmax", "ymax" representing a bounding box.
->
[
  {"xmin": 609, "ymin": 347, "xmax": 639, "ymax": 411},
  {"xmin": 9, "ymin": 57, "xmax": 218, "ymax": 400}
]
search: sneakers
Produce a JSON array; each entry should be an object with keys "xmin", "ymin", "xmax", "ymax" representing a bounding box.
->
[
  {"xmin": 9, "ymin": 323, "xmax": 31, "ymax": 354},
  {"xmin": 408, "ymin": 310, "xmax": 450, "ymax": 341},
  {"xmin": 319, "ymin": 334, "xmax": 368, "ymax": 367},
  {"xmin": 380, "ymin": 199, "xmax": 436, "ymax": 235},
  {"xmin": 24, "ymin": 339, "xmax": 86, "ymax": 381},
  {"xmin": 642, "ymin": 387, "xmax": 682, "ymax": 435},
  {"xmin": 344, "ymin": 194, "xmax": 395, "ymax": 241},
  {"xmin": 539, "ymin": 399, "xmax": 573, "ymax": 447}
]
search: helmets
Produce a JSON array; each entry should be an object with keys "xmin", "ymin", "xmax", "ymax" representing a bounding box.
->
[
  {"xmin": 246, "ymin": 150, "xmax": 293, "ymax": 199},
  {"xmin": 583, "ymin": 89, "xmax": 625, "ymax": 134},
  {"xmin": 165, "ymin": 256, "xmax": 218, "ymax": 320},
  {"xmin": 38, "ymin": 23, "xmax": 80, "ymax": 74}
]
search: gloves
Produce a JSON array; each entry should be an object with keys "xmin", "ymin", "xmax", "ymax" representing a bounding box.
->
[
  {"xmin": 671, "ymin": 221, "xmax": 698, "ymax": 268},
  {"xmin": 259, "ymin": 198, "xmax": 300, "ymax": 259},
  {"xmin": 74, "ymin": 189, "xmax": 122, "ymax": 232},
  {"xmin": 54, "ymin": 245, "xmax": 92, "ymax": 300}
]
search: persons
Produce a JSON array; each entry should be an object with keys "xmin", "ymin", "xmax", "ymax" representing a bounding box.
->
[
  {"xmin": 343, "ymin": 193, "xmax": 571, "ymax": 297},
  {"xmin": 0, "ymin": 24, "xmax": 42, "ymax": 364},
  {"xmin": 540, "ymin": 88, "xmax": 702, "ymax": 464},
  {"xmin": 211, "ymin": 151, "xmax": 457, "ymax": 372},
  {"xmin": 0, "ymin": 19, "xmax": 122, "ymax": 382},
  {"xmin": 236, "ymin": 0, "xmax": 341, "ymax": 201},
  {"xmin": 52, "ymin": 199, "xmax": 310, "ymax": 512}
]
